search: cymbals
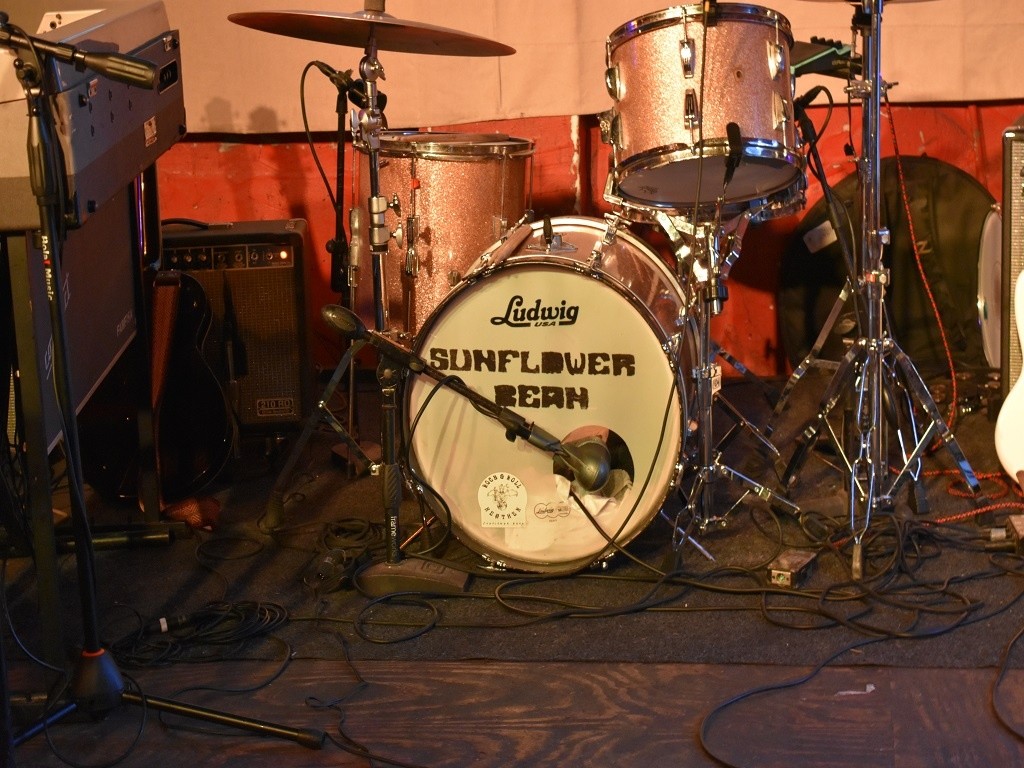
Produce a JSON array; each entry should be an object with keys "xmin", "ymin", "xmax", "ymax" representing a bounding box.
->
[{"xmin": 227, "ymin": 9, "xmax": 517, "ymax": 58}]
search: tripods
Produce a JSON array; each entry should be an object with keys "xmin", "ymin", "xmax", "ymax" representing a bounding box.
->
[
  {"xmin": 656, "ymin": 0, "xmax": 994, "ymax": 584},
  {"xmin": 0, "ymin": 26, "xmax": 420, "ymax": 768}
]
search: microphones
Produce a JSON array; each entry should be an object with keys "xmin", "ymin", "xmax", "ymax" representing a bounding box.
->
[
  {"xmin": 220, "ymin": 280, "xmax": 251, "ymax": 378},
  {"xmin": 793, "ymin": 87, "xmax": 821, "ymax": 122},
  {"xmin": 316, "ymin": 60, "xmax": 387, "ymax": 113},
  {"xmin": 552, "ymin": 437, "xmax": 612, "ymax": 493}
]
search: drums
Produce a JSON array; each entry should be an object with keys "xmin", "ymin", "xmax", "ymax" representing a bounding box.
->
[
  {"xmin": 399, "ymin": 216, "xmax": 701, "ymax": 575},
  {"xmin": 604, "ymin": 1, "xmax": 809, "ymax": 221},
  {"xmin": 352, "ymin": 131, "xmax": 536, "ymax": 371},
  {"xmin": 975, "ymin": 200, "xmax": 1003, "ymax": 372}
]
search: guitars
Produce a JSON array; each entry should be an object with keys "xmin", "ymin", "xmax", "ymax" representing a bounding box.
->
[{"xmin": 994, "ymin": 269, "xmax": 1024, "ymax": 485}]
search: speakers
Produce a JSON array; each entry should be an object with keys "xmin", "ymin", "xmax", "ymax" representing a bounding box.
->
[
  {"xmin": 1001, "ymin": 126, "xmax": 1024, "ymax": 398},
  {"xmin": 157, "ymin": 219, "xmax": 305, "ymax": 435}
]
